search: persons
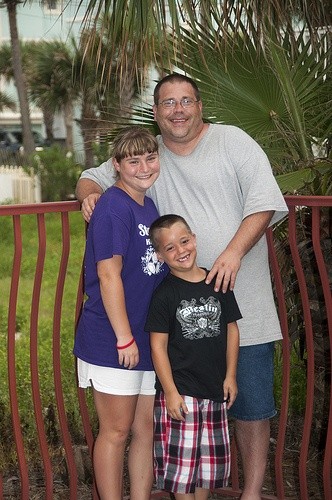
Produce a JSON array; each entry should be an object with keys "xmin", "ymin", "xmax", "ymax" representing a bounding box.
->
[
  {"xmin": 74, "ymin": 73, "xmax": 290, "ymax": 500},
  {"xmin": 71, "ymin": 127, "xmax": 168, "ymax": 500},
  {"xmin": 138, "ymin": 215, "xmax": 244, "ymax": 500}
]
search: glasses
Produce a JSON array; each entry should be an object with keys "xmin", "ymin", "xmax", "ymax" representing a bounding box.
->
[{"xmin": 156, "ymin": 97, "xmax": 200, "ymax": 108}]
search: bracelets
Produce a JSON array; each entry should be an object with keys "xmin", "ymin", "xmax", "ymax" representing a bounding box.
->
[{"xmin": 116, "ymin": 337, "xmax": 135, "ymax": 350}]
